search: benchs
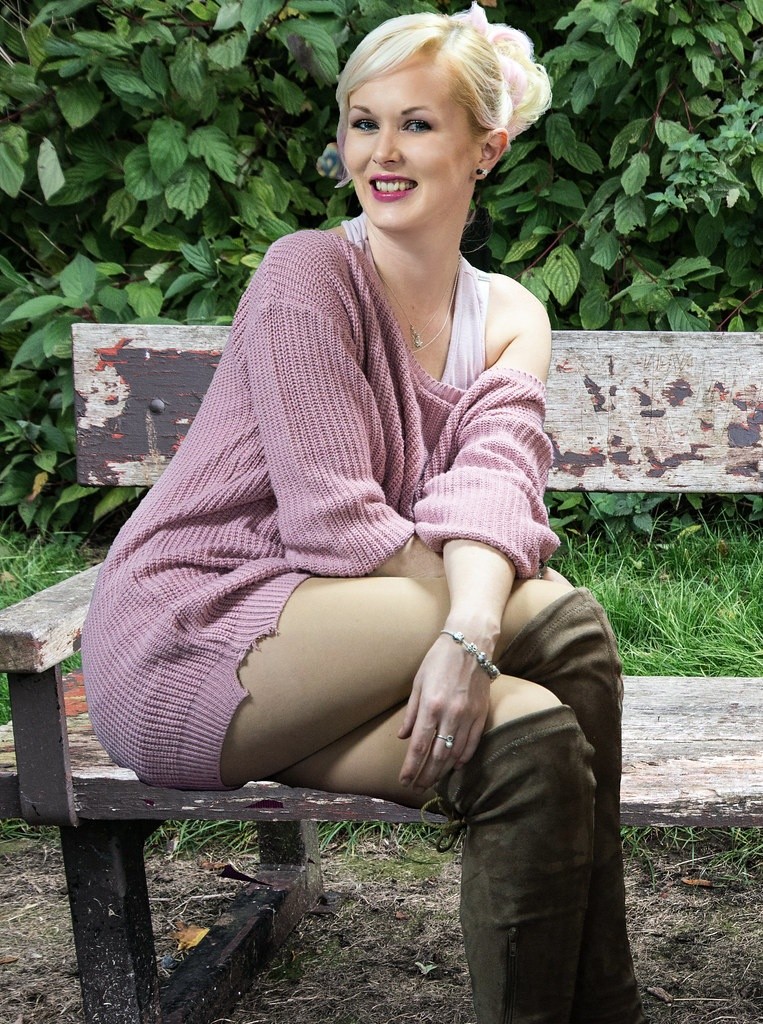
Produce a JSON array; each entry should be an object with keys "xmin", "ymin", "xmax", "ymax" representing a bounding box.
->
[{"xmin": 0, "ymin": 324, "xmax": 763, "ymax": 1024}]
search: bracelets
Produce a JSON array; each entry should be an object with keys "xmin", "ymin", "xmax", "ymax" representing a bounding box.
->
[{"xmin": 441, "ymin": 630, "xmax": 499, "ymax": 678}]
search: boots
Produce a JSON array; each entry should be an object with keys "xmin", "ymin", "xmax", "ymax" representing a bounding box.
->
[
  {"xmin": 500, "ymin": 587, "xmax": 649, "ymax": 1024},
  {"xmin": 419, "ymin": 702, "xmax": 597, "ymax": 1024}
]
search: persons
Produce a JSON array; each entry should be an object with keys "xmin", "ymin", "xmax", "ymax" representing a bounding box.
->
[{"xmin": 85, "ymin": 14, "xmax": 640, "ymax": 1024}]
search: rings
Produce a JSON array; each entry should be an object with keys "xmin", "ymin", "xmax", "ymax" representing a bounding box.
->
[{"xmin": 437, "ymin": 735, "xmax": 454, "ymax": 747}]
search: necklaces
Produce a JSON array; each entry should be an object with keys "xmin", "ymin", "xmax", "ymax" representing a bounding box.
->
[{"xmin": 378, "ymin": 255, "xmax": 461, "ymax": 354}]
